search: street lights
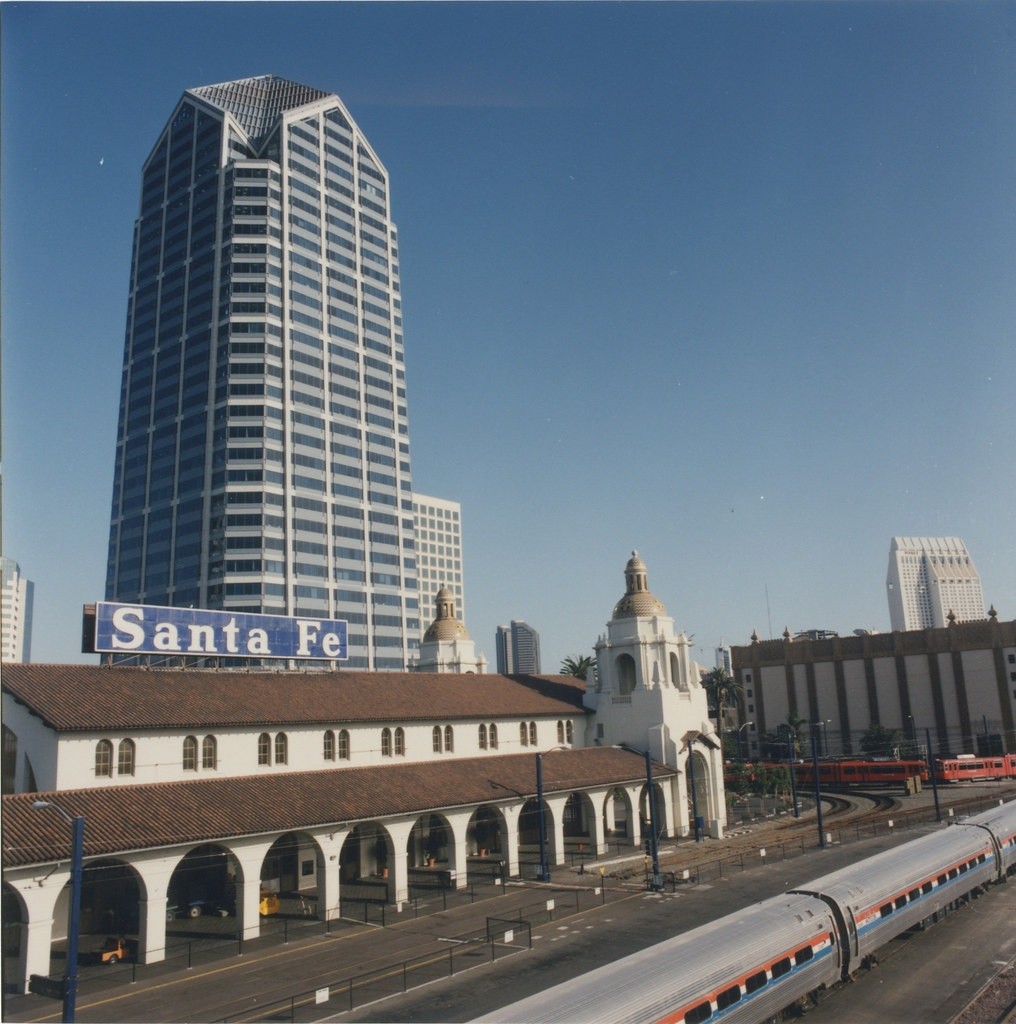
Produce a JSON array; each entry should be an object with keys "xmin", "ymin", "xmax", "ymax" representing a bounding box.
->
[
  {"xmin": 785, "ymin": 728, "xmax": 800, "ymax": 818},
  {"xmin": 678, "ymin": 729, "xmax": 721, "ymax": 842},
  {"xmin": 535, "ymin": 742, "xmax": 572, "ymax": 881},
  {"xmin": 816, "ymin": 719, "xmax": 832, "ymax": 755},
  {"xmin": 611, "ymin": 740, "xmax": 665, "ymax": 892},
  {"xmin": 903, "ymin": 712, "xmax": 920, "ymax": 754},
  {"xmin": 724, "ymin": 721, "xmax": 754, "ymax": 763}
]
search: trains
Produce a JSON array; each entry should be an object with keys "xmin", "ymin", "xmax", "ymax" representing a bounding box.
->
[
  {"xmin": 724, "ymin": 752, "xmax": 1016, "ymax": 788},
  {"xmin": 461, "ymin": 798, "xmax": 1015, "ymax": 1024}
]
